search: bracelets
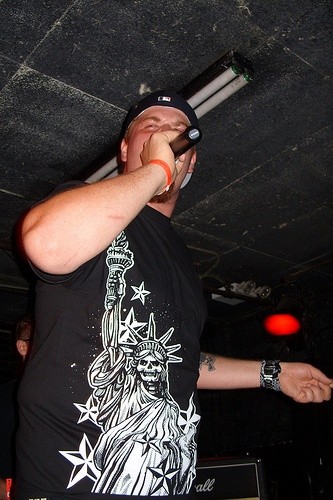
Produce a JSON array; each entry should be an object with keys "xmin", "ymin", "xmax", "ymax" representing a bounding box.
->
[{"xmin": 146, "ymin": 159, "xmax": 172, "ymax": 196}]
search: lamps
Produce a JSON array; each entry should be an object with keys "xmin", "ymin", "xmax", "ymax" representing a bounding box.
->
[{"xmin": 83, "ymin": 49, "xmax": 254, "ymax": 184}]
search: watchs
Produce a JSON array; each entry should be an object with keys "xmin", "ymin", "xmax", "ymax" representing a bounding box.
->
[{"xmin": 260, "ymin": 359, "xmax": 281, "ymax": 393}]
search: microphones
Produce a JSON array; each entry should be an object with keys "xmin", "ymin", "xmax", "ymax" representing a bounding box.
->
[{"xmin": 169, "ymin": 126, "xmax": 203, "ymax": 162}]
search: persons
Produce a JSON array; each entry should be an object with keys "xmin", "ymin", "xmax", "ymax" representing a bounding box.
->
[{"xmin": 11, "ymin": 89, "xmax": 333, "ymax": 499}]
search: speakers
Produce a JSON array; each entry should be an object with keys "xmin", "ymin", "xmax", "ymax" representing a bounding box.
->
[{"xmin": 189, "ymin": 456, "xmax": 269, "ymax": 500}]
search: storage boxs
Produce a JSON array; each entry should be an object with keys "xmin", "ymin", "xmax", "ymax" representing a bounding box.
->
[{"xmin": 196, "ymin": 461, "xmax": 261, "ymax": 500}]
